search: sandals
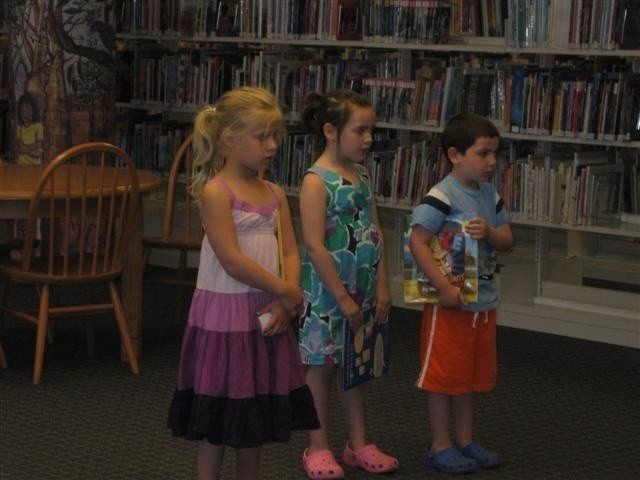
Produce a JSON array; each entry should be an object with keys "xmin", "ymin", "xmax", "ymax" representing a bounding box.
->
[
  {"xmin": 342, "ymin": 442, "xmax": 399, "ymax": 474},
  {"xmin": 422, "ymin": 441, "xmax": 500, "ymax": 474},
  {"xmin": 303, "ymin": 447, "xmax": 345, "ymax": 480}
]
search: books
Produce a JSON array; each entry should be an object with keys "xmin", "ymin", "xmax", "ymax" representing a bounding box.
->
[
  {"xmin": 106, "ymin": 2, "xmax": 640, "ymax": 228},
  {"xmin": 404, "ymin": 214, "xmax": 478, "ymax": 303}
]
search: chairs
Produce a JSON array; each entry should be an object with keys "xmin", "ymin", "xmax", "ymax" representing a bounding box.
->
[
  {"xmin": 142, "ymin": 133, "xmax": 204, "ymax": 326},
  {"xmin": 1, "ymin": 142, "xmax": 140, "ymax": 387}
]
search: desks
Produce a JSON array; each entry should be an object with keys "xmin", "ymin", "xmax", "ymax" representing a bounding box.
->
[{"xmin": 0, "ymin": 163, "xmax": 162, "ymax": 361}]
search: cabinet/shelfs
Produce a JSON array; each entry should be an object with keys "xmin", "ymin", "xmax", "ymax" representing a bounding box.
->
[{"xmin": 111, "ymin": 0, "xmax": 640, "ymax": 349}]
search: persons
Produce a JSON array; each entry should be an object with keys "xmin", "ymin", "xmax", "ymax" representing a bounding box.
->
[
  {"xmin": 296, "ymin": 90, "xmax": 399, "ymax": 477},
  {"xmin": 413, "ymin": 114, "xmax": 513, "ymax": 475},
  {"xmin": 8, "ymin": 91, "xmax": 45, "ymax": 260},
  {"xmin": 166, "ymin": 85, "xmax": 323, "ymax": 478}
]
